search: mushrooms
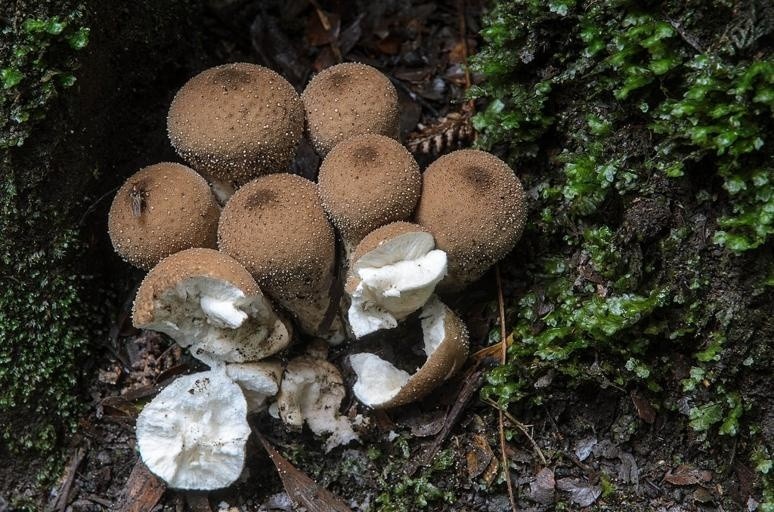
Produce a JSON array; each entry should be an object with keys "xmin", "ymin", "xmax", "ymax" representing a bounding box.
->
[{"xmin": 107, "ymin": 61, "xmax": 528, "ymax": 495}]
